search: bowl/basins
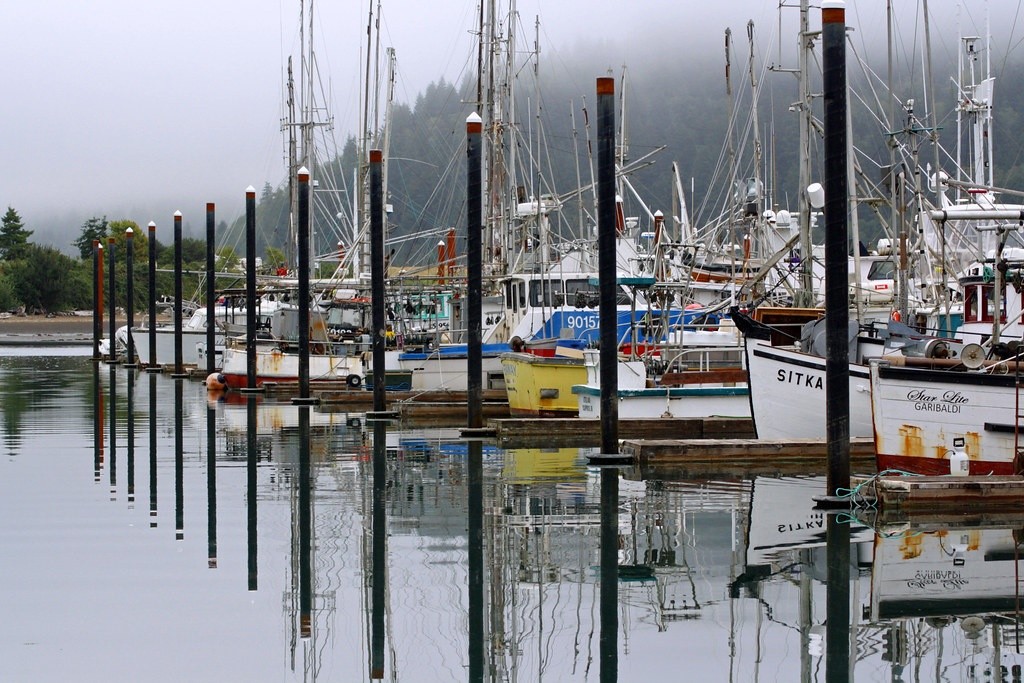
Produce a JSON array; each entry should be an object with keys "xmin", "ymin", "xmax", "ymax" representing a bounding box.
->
[{"xmin": 524, "ymin": 340, "xmax": 558, "ymax": 357}]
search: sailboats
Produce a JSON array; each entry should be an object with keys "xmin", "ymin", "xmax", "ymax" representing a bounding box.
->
[
  {"xmin": 130, "ymin": 0, "xmax": 1024, "ymax": 507},
  {"xmin": 216, "ymin": 400, "xmax": 1023, "ymax": 683}
]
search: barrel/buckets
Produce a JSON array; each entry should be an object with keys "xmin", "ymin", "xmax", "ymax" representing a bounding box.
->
[{"xmin": 806, "ymin": 182, "xmax": 827, "ymax": 209}]
